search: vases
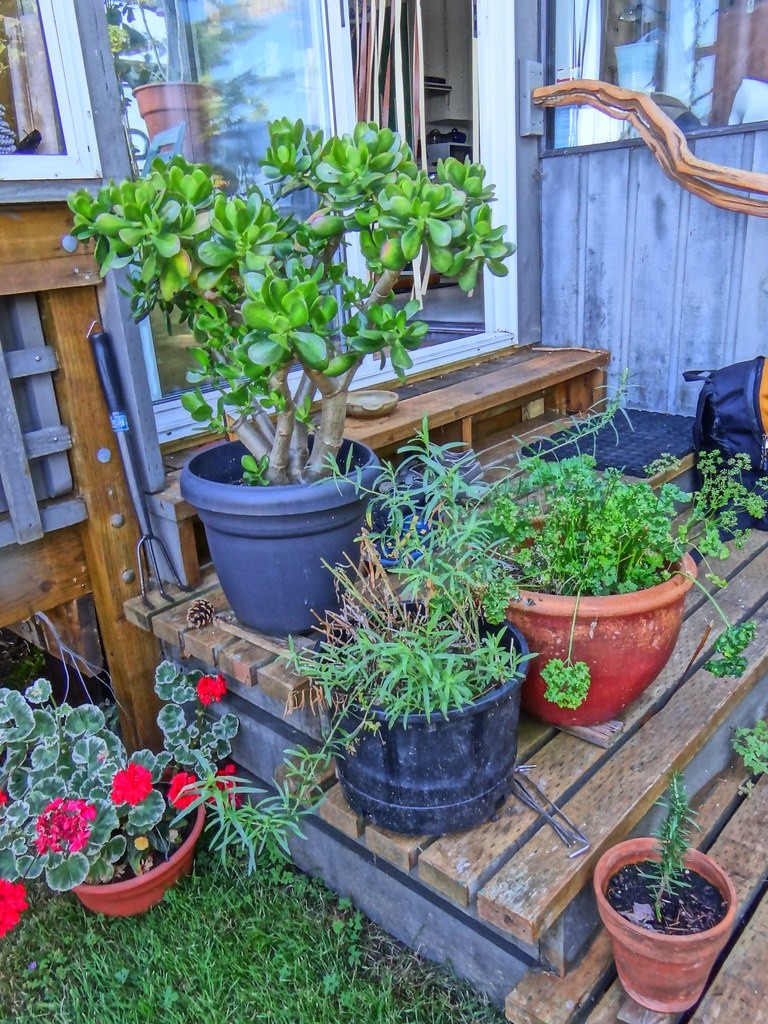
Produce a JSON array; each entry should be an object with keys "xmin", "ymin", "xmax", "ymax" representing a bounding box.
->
[{"xmin": 70, "ymin": 768, "xmax": 205, "ymax": 916}]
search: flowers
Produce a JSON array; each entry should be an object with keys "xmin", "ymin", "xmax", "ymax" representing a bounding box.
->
[{"xmin": 0, "ymin": 661, "xmax": 241, "ymax": 936}]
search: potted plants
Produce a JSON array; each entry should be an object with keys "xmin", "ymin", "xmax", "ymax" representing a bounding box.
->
[
  {"xmin": 457, "ymin": 446, "xmax": 767, "ymax": 726},
  {"xmin": 66, "ymin": 113, "xmax": 518, "ymax": 637},
  {"xmin": 169, "ymin": 417, "xmax": 538, "ymax": 876},
  {"xmin": 593, "ymin": 768, "xmax": 738, "ymax": 1013},
  {"xmin": 0, "ymin": 0, "xmax": 274, "ymax": 162}
]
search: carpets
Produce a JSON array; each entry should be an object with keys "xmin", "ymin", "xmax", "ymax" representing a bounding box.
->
[{"xmin": 520, "ymin": 406, "xmax": 695, "ymax": 479}]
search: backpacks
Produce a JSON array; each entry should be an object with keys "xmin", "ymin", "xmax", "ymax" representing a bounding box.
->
[{"xmin": 684, "ymin": 356, "xmax": 768, "ymax": 540}]
